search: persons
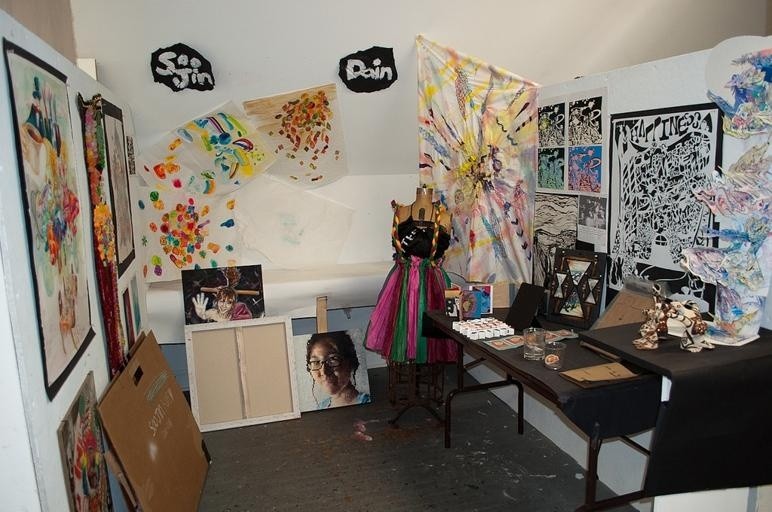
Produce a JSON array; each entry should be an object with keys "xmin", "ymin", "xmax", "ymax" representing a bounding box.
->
[
  {"xmin": 192, "ymin": 267, "xmax": 253, "ymax": 323},
  {"xmin": 305, "ymin": 330, "xmax": 367, "ymax": 410},
  {"xmin": 363, "ymin": 187, "xmax": 461, "ymax": 364}
]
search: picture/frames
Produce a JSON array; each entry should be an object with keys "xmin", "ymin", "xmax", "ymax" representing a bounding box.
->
[
  {"xmin": 546, "ymin": 248, "xmax": 608, "ymax": 332},
  {"xmin": 183, "ymin": 315, "xmax": 302, "ymax": 433}
]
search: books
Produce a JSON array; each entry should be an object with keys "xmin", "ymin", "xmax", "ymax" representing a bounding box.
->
[{"xmin": 558, "ymin": 362, "xmax": 640, "ymax": 389}]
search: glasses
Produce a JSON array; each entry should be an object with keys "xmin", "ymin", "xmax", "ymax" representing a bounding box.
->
[{"xmin": 307, "ymin": 358, "xmax": 346, "ymax": 371}]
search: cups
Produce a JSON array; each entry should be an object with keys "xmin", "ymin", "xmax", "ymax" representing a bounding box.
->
[
  {"xmin": 543, "ymin": 342, "xmax": 566, "ymax": 371},
  {"xmin": 523, "ymin": 328, "xmax": 546, "ymax": 361}
]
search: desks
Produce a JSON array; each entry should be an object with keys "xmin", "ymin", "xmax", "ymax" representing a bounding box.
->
[{"xmin": 423, "ymin": 307, "xmax": 772, "ymax": 512}]
